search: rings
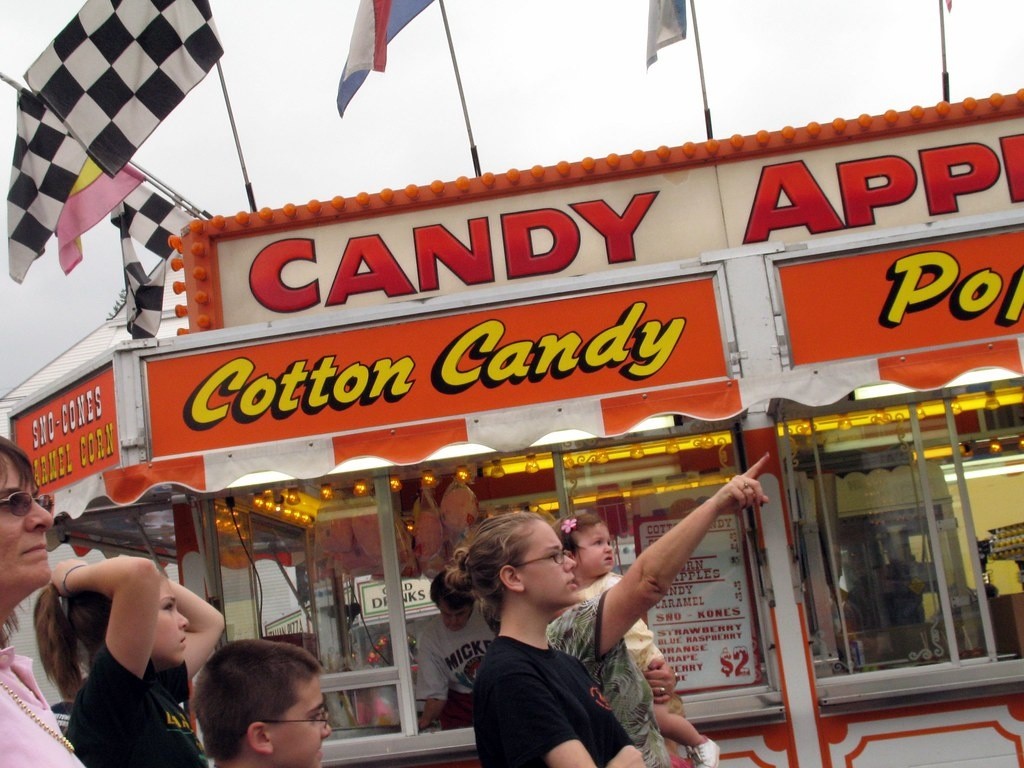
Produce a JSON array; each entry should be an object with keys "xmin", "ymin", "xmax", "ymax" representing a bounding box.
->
[
  {"xmin": 660, "ymin": 686, "xmax": 665, "ymax": 695},
  {"xmin": 743, "ymin": 483, "xmax": 749, "ymax": 491}
]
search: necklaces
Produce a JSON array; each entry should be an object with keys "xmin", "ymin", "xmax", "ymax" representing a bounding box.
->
[{"xmin": 0, "ymin": 680, "xmax": 74, "ymax": 753}]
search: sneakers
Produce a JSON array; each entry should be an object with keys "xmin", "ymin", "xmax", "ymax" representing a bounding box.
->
[{"xmin": 686, "ymin": 735, "xmax": 720, "ymax": 768}]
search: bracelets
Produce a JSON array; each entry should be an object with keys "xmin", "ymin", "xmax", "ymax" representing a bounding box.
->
[{"xmin": 63, "ymin": 563, "xmax": 88, "ymax": 596}]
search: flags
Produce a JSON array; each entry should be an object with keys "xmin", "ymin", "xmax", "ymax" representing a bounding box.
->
[
  {"xmin": 7, "ymin": 1, "xmax": 225, "ymax": 340},
  {"xmin": 336, "ymin": 0, "xmax": 433, "ymax": 119},
  {"xmin": 646, "ymin": 0, "xmax": 690, "ymax": 76}
]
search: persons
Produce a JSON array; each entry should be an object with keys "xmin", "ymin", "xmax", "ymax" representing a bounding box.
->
[
  {"xmin": 480, "ymin": 449, "xmax": 770, "ymax": 768},
  {"xmin": 36, "ymin": 553, "xmax": 227, "ymax": 768},
  {"xmin": 556, "ymin": 512, "xmax": 720, "ymax": 768},
  {"xmin": 443, "ymin": 510, "xmax": 649, "ymax": 768},
  {"xmin": 0, "ymin": 435, "xmax": 86, "ymax": 768},
  {"xmin": 194, "ymin": 638, "xmax": 335, "ymax": 768},
  {"xmin": 419, "ymin": 566, "xmax": 496, "ymax": 732}
]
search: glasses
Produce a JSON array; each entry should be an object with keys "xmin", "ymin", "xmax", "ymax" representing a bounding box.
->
[
  {"xmin": 0, "ymin": 491, "xmax": 54, "ymax": 516},
  {"xmin": 261, "ymin": 708, "xmax": 327, "ymax": 729},
  {"xmin": 514, "ymin": 550, "xmax": 569, "ymax": 568}
]
